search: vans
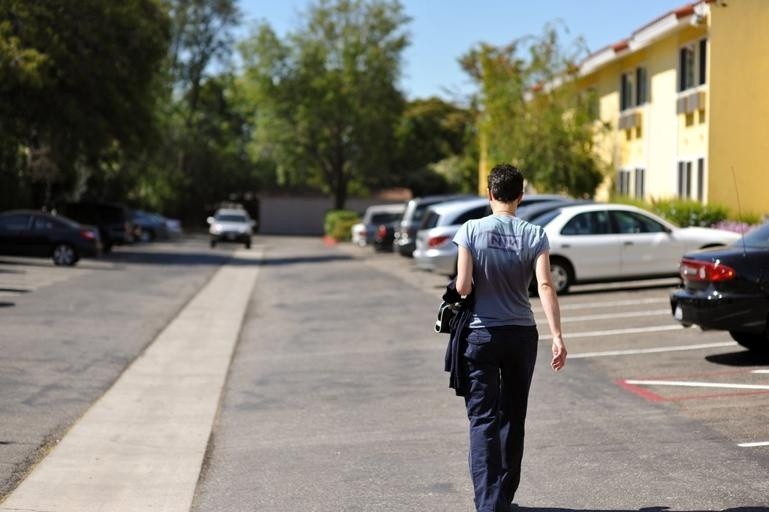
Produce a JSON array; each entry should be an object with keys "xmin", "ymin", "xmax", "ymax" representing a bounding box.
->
[{"xmin": 40, "ymin": 202, "xmax": 136, "ymax": 254}]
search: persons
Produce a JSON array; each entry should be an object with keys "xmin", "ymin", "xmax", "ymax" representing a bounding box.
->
[{"xmin": 452, "ymin": 161, "xmax": 567, "ymax": 511}]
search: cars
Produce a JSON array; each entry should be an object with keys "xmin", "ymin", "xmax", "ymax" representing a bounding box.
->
[
  {"xmin": 525, "ymin": 204, "xmax": 743, "ymax": 295},
  {"xmin": 0, "ymin": 209, "xmax": 101, "ymax": 265},
  {"xmin": 205, "ymin": 208, "xmax": 258, "ymax": 248},
  {"xmin": 352, "ymin": 194, "xmax": 597, "ymax": 281},
  {"xmin": 671, "ymin": 218, "xmax": 769, "ymax": 350},
  {"xmin": 127, "ymin": 207, "xmax": 184, "ymax": 243}
]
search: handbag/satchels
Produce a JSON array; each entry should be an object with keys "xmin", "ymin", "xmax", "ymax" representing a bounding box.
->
[{"xmin": 432, "ymin": 297, "xmax": 476, "ymax": 335}]
